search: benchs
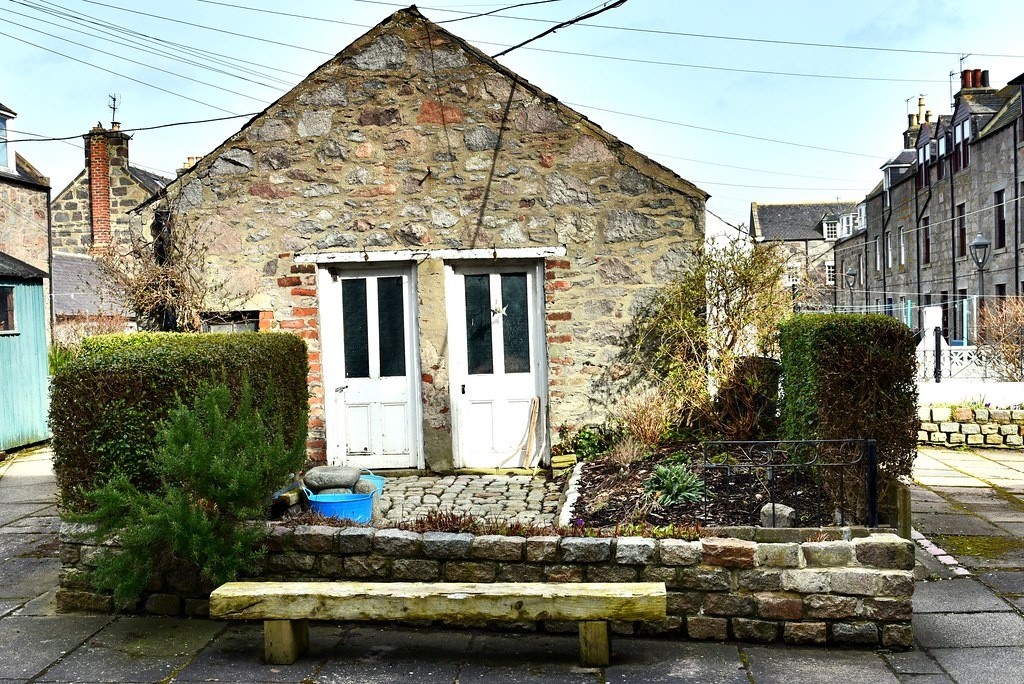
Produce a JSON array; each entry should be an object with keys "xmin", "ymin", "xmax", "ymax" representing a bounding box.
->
[{"xmin": 207, "ymin": 581, "xmax": 670, "ymax": 669}]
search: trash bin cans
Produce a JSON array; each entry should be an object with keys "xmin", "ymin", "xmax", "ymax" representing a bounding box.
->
[{"xmin": 714, "ymin": 356, "xmax": 783, "ymax": 417}]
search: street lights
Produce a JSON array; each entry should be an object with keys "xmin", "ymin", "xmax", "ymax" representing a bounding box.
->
[
  {"xmin": 841, "ymin": 266, "xmax": 857, "ymax": 316},
  {"xmin": 969, "ymin": 232, "xmax": 994, "ymax": 344}
]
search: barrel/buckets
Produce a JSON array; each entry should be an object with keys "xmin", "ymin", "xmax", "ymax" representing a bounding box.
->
[
  {"xmin": 359, "ymin": 475, "xmax": 386, "ymax": 502},
  {"xmin": 302, "ymin": 486, "xmax": 373, "ymax": 524},
  {"xmin": 950, "ymin": 340, "xmax": 963, "ymax": 346}
]
